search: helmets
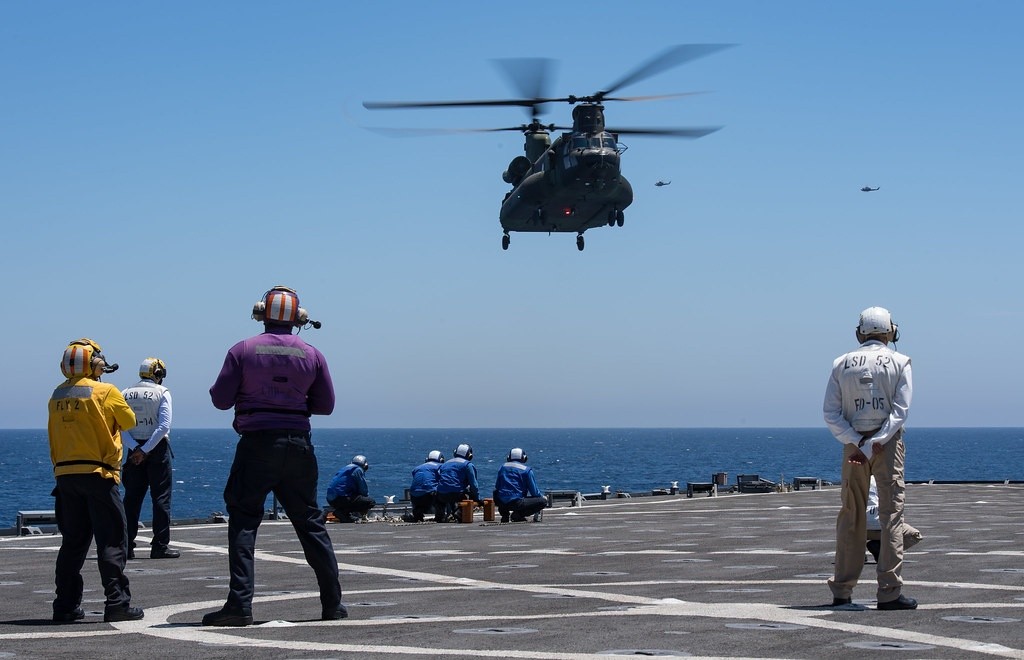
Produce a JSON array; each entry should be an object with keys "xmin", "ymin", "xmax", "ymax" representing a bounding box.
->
[
  {"xmin": 140, "ymin": 358, "xmax": 166, "ymax": 383},
  {"xmin": 263, "ymin": 290, "xmax": 298, "ymax": 327},
  {"xmin": 859, "ymin": 307, "xmax": 893, "ymax": 335},
  {"xmin": 456, "ymin": 443, "xmax": 472, "ymax": 457},
  {"xmin": 61, "ymin": 339, "xmax": 101, "ymax": 377},
  {"xmin": 429, "ymin": 450, "xmax": 444, "ymax": 461},
  {"xmin": 353, "ymin": 455, "xmax": 367, "ymax": 466},
  {"xmin": 510, "ymin": 448, "xmax": 525, "ymax": 460}
]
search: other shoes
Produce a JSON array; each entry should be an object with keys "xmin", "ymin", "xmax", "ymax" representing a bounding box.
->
[
  {"xmin": 865, "ymin": 542, "xmax": 880, "ymax": 562},
  {"xmin": 501, "ymin": 517, "xmax": 509, "ymax": 522},
  {"xmin": 510, "ymin": 517, "xmax": 526, "ymax": 522},
  {"xmin": 334, "ymin": 510, "xmax": 358, "ymax": 522}
]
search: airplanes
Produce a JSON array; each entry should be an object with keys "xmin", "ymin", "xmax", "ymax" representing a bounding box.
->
[
  {"xmin": 860, "ymin": 186, "xmax": 880, "ymax": 192},
  {"xmin": 655, "ymin": 180, "xmax": 672, "ymax": 187}
]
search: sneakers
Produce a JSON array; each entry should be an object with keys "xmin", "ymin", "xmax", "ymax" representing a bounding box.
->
[
  {"xmin": 321, "ymin": 604, "xmax": 347, "ymax": 620},
  {"xmin": 129, "ymin": 551, "xmax": 135, "ymax": 557},
  {"xmin": 151, "ymin": 550, "xmax": 179, "ymax": 558},
  {"xmin": 102, "ymin": 606, "xmax": 144, "ymax": 621},
  {"xmin": 53, "ymin": 608, "xmax": 84, "ymax": 622},
  {"xmin": 834, "ymin": 597, "xmax": 851, "ymax": 604},
  {"xmin": 878, "ymin": 595, "xmax": 917, "ymax": 609},
  {"xmin": 202, "ymin": 602, "xmax": 253, "ymax": 626}
]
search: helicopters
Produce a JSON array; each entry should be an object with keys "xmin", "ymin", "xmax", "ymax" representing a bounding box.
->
[{"xmin": 362, "ymin": 41, "xmax": 746, "ymax": 251}]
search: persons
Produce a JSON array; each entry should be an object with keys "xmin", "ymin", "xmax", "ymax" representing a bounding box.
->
[
  {"xmin": 492, "ymin": 448, "xmax": 547, "ymax": 522},
  {"xmin": 436, "ymin": 443, "xmax": 479, "ymax": 521},
  {"xmin": 327, "ymin": 456, "xmax": 376, "ymax": 523},
  {"xmin": 409, "ymin": 450, "xmax": 445, "ymax": 521},
  {"xmin": 49, "ymin": 339, "xmax": 144, "ymax": 621},
  {"xmin": 203, "ymin": 292, "xmax": 348, "ymax": 623},
  {"xmin": 823, "ymin": 307, "xmax": 919, "ymax": 610},
  {"xmin": 121, "ymin": 357, "xmax": 180, "ymax": 558},
  {"xmin": 866, "ymin": 475, "xmax": 923, "ymax": 563}
]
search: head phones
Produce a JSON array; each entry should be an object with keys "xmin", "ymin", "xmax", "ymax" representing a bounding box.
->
[
  {"xmin": 453, "ymin": 444, "xmax": 473, "ymax": 461},
  {"xmin": 69, "ymin": 338, "xmax": 106, "ymax": 377},
  {"xmin": 155, "ymin": 363, "xmax": 163, "ymax": 379},
  {"xmin": 856, "ymin": 324, "xmax": 897, "ymax": 344},
  {"xmin": 350, "ymin": 460, "xmax": 368, "ymax": 471},
  {"xmin": 253, "ymin": 286, "xmax": 308, "ymax": 327},
  {"xmin": 425, "ymin": 457, "xmax": 445, "ymax": 463},
  {"xmin": 507, "ymin": 455, "xmax": 528, "ymax": 462}
]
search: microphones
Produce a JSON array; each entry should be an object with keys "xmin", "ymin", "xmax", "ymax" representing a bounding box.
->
[
  {"xmin": 103, "ymin": 363, "xmax": 118, "ymax": 373},
  {"xmin": 308, "ymin": 320, "xmax": 321, "ymax": 329}
]
